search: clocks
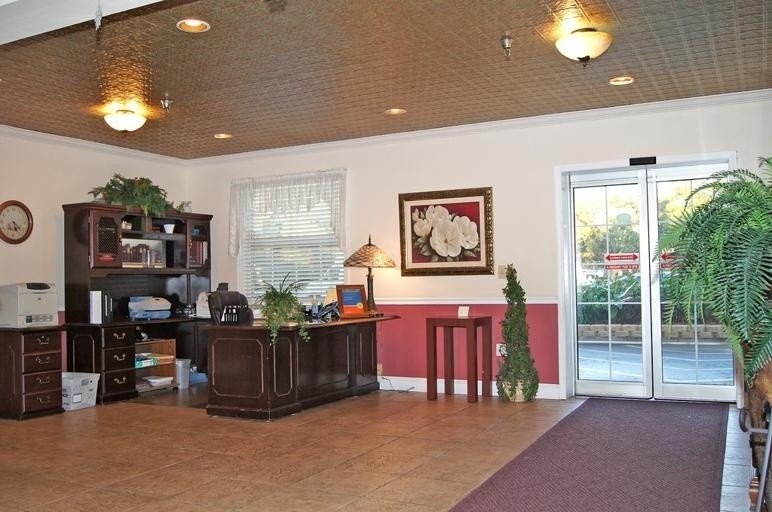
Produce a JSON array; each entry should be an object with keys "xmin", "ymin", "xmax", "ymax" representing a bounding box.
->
[{"xmin": 0, "ymin": 198, "xmax": 33, "ymax": 246}]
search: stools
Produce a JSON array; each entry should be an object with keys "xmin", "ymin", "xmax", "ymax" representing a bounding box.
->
[{"xmin": 427, "ymin": 316, "xmax": 494, "ymax": 402}]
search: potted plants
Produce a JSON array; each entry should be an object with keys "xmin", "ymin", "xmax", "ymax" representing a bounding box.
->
[
  {"xmin": 659, "ymin": 151, "xmax": 772, "ymax": 388},
  {"xmin": 495, "ymin": 262, "xmax": 542, "ymax": 404},
  {"xmin": 253, "ymin": 270, "xmax": 317, "ymax": 349}
]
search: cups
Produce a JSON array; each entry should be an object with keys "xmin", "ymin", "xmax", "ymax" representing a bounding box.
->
[
  {"xmin": 141, "ymin": 249, "xmax": 156, "ymax": 269},
  {"xmin": 164, "ymin": 224, "xmax": 175, "ymax": 234}
]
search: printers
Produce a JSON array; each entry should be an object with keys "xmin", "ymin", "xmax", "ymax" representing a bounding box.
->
[{"xmin": 0, "ymin": 282, "xmax": 59, "ymax": 328}]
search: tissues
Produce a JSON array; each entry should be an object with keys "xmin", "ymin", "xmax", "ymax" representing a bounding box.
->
[{"xmin": 196, "ymin": 292, "xmax": 211, "ymax": 318}]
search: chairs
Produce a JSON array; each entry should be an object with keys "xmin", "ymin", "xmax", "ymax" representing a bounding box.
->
[{"xmin": 206, "ymin": 289, "xmax": 255, "ymax": 325}]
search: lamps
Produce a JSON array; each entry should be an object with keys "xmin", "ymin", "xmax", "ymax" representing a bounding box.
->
[
  {"xmin": 90, "ymin": 49, "xmax": 157, "ymax": 133},
  {"xmin": 341, "ymin": 235, "xmax": 398, "ymax": 321},
  {"xmin": 556, "ymin": 26, "xmax": 614, "ymax": 64}
]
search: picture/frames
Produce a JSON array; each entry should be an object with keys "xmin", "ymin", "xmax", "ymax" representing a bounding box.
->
[
  {"xmin": 395, "ymin": 187, "xmax": 496, "ymax": 278},
  {"xmin": 336, "ymin": 282, "xmax": 371, "ymax": 320}
]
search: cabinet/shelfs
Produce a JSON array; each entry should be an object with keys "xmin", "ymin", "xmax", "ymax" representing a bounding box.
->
[
  {"xmin": 58, "ymin": 202, "xmax": 214, "ymax": 404},
  {"xmin": 1, "ymin": 327, "xmax": 67, "ymax": 420}
]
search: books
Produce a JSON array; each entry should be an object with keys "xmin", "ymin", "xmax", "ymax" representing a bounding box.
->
[
  {"xmin": 189, "ymin": 240, "xmax": 208, "ymax": 268},
  {"xmin": 139, "ymin": 376, "xmax": 174, "ymax": 392},
  {"xmin": 134, "ymin": 352, "xmax": 175, "ymax": 368},
  {"xmin": 122, "ymin": 242, "xmax": 162, "ymax": 267}
]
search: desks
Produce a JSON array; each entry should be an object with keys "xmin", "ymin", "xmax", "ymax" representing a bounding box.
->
[{"xmin": 208, "ymin": 314, "xmax": 400, "ymax": 420}]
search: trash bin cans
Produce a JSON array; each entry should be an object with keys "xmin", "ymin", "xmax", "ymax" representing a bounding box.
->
[{"xmin": 176, "ymin": 358, "xmax": 191, "ymax": 389}]
